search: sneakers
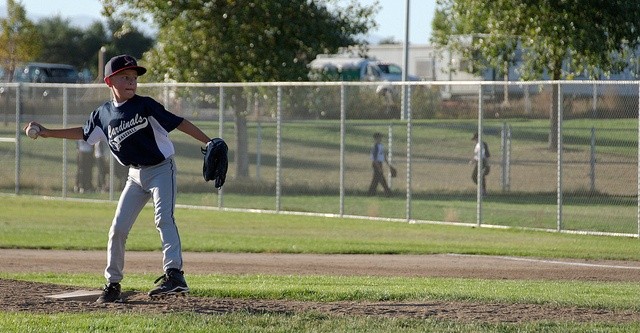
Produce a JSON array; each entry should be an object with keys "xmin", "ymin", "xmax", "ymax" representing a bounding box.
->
[
  {"xmin": 148, "ymin": 268, "xmax": 189, "ymax": 297},
  {"xmin": 96, "ymin": 282, "xmax": 122, "ymax": 304}
]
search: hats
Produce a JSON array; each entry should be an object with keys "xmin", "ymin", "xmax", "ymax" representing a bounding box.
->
[{"xmin": 105, "ymin": 55, "xmax": 147, "ymax": 77}]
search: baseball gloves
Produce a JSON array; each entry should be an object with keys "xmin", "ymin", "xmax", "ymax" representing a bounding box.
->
[{"xmin": 201, "ymin": 137, "xmax": 228, "ymax": 190}]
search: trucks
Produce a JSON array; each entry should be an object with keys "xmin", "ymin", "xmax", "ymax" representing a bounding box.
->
[{"xmin": 310, "ymin": 54, "xmax": 421, "ymax": 106}]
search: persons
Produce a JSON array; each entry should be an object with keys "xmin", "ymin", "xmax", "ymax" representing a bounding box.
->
[
  {"xmin": 30, "ymin": 54, "xmax": 212, "ymax": 304},
  {"xmin": 95, "ymin": 141, "xmax": 108, "ymax": 191},
  {"xmin": 472, "ymin": 131, "xmax": 490, "ymax": 190},
  {"xmin": 370, "ymin": 132, "xmax": 397, "ymax": 192},
  {"xmin": 77, "ymin": 139, "xmax": 94, "ymax": 192}
]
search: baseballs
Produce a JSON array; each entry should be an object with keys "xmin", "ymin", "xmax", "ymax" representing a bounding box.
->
[{"xmin": 26, "ymin": 125, "xmax": 40, "ymax": 139}]
